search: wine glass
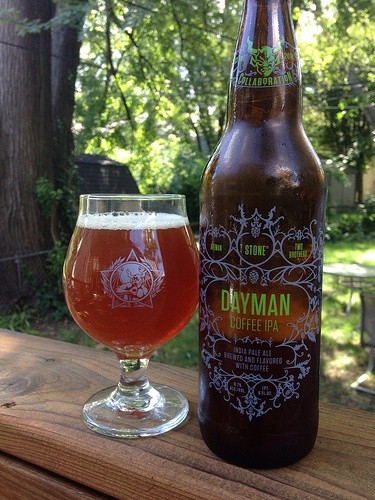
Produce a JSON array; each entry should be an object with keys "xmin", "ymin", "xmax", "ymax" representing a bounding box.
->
[{"xmin": 62, "ymin": 194, "xmax": 196, "ymax": 438}]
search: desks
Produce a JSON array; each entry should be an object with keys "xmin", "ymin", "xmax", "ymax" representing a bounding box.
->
[{"xmin": 322, "ymin": 264, "xmax": 375, "ymax": 315}]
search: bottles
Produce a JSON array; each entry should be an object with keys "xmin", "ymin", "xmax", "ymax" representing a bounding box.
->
[{"xmin": 197, "ymin": 0, "xmax": 326, "ymax": 471}]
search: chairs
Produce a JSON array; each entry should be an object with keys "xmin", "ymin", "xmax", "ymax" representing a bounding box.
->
[{"xmin": 351, "ymin": 292, "xmax": 375, "ymax": 394}]
